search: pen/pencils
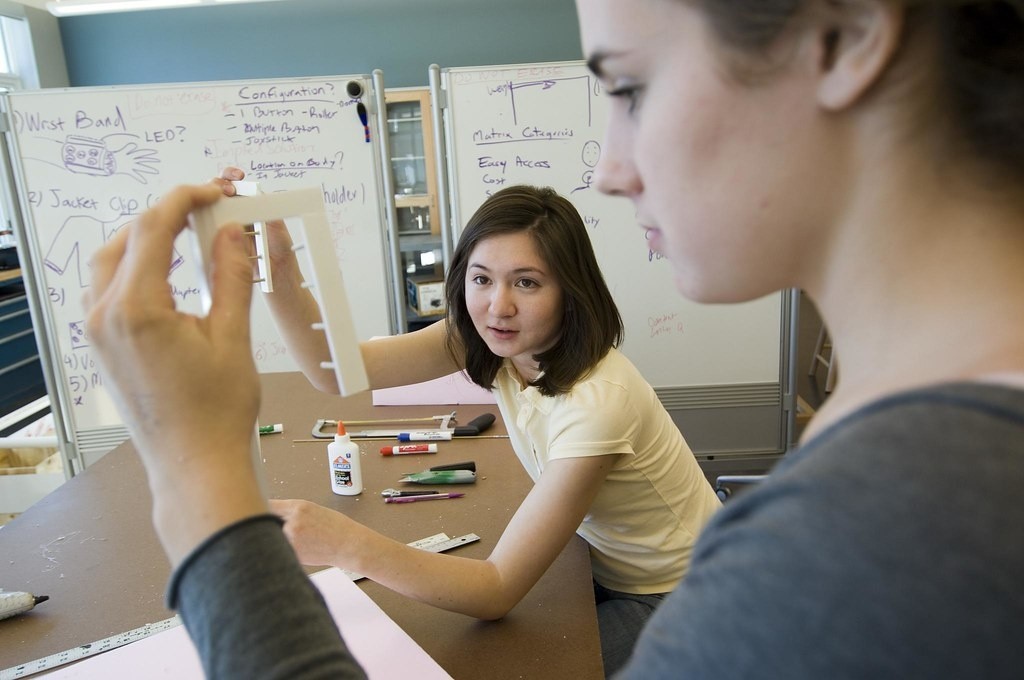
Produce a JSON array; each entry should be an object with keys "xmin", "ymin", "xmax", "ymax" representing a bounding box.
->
[{"xmin": 384, "ymin": 493, "xmax": 466, "ymax": 504}]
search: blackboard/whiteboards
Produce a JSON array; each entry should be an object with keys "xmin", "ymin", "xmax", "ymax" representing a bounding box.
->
[
  {"xmin": 440, "ymin": 61, "xmax": 790, "ymax": 391},
  {"xmin": 0, "ymin": 73, "xmax": 398, "ymax": 439}
]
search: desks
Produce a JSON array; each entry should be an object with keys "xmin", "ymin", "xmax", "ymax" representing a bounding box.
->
[{"xmin": 0, "ymin": 371, "xmax": 607, "ymax": 680}]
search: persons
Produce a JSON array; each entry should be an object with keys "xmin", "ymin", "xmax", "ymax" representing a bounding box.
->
[
  {"xmin": 209, "ymin": 165, "xmax": 728, "ymax": 680},
  {"xmin": 84, "ymin": 2, "xmax": 1024, "ymax": 680}
]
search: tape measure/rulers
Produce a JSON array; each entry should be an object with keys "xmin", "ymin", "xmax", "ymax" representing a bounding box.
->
[{"xmin": 0, "ymin": 531, "xmax": 482, "ymax": 680}]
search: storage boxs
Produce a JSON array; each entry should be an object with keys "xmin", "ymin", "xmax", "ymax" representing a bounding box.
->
[{"xmin": 407, "ymin": 276, "xmax": 447, "ymax": 317}]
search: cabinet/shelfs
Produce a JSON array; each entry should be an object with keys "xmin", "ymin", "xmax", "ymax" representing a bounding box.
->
[{"xmin": 384, "ymin": 86, "xmax": 449, "ymax": 323}]
